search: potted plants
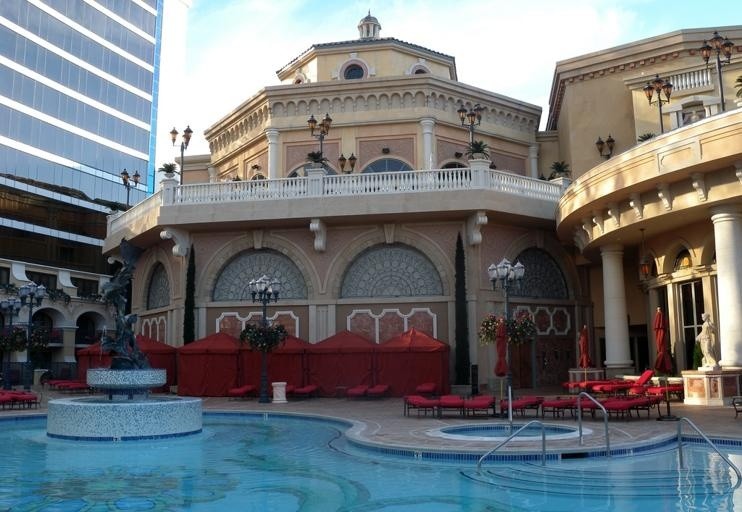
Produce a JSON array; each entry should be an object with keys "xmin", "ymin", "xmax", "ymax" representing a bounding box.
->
[
  {"xmin": 307, "ymin": 150, "xmax": 330, "ymax": 169},
  {"xmin": 156, "ymin": 163, "xmax": 183, "ymax": 179},
  {"xmin": 548, "ymin": 158, "xmax": 573, "ymax": 180},
  {"xmin": 466, "ymin": 139, "xmax": 492, "ymax": 160}
]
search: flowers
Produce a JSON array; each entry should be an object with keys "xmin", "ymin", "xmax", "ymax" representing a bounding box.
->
[
  {"xmin": 478, "ymin": 307, "xmax": 538, "ymax": 348},
  {"xmin": 1, "ymin": 322, "xmax": 51, "ymax": 359},
  {"xmin": 236, "ymin": 321, "xmax": 291, "ymax": 352}
]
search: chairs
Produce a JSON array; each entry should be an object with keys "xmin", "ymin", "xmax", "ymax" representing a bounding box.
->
[
  {"xmin": 563, "ymin": 379, "xmax": 685, "ymax": 401},
  {"xmin": 404, "ymin": 393, "xmax": 671, "ymax": 421},
  {"xmin": 230, "ymin": 381, "xmax": 440, "ymax": 400},
  {"xmin": 1, "ymin": 378, "xmax": 95, "ymax": 409}
]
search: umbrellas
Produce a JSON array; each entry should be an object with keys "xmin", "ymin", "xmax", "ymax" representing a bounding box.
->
[
  {"xmin": 578, "ymin": 325, "xmax": 592, "ymax": 368},
  {"xmin": 654, "ymin": 307, "xmax": 675, "ymax": 374},
  {"xmin": 493, "ymin": 318, "xmax": 509, "ymax": 379}
]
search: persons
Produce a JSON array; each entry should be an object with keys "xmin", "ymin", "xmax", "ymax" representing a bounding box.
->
[{"xmin": 693, "ymin": 314, "xmax": 721, "ymax": 369}]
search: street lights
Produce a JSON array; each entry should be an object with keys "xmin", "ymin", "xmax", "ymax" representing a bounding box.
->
[
  {"xmin": 640, "ymin": 72, "xmax": 674, "ymax": 135},
  {"xmin": 246, "ymin": 273, "xmax": 285, "ymax": 405},
  {"xmin": 18, "ymin": 281, "xmax": 48, "ymax": 392},
  {"xmin": 456, "ymin": 103, "xmax": 483, "ymax": 154},
  {"xmin": 0, "ymin": 296, "xmax": 23, "ymax": 390},
  {"xmin": 484, "ymin": 257, "xmax": 531, "ymax": 402},
  {"xmin": 119, "ymin": 166, "xmax": 140, "ymax": 213},
  {"xmin": 699, "ymin": 26, "xmax": 733, "ymax": 112},
  {"xmin": 169, "ymin": 124, "xmax": 195, "ymax": 200},
  {"xmin": 306, "ymin": 112, "xmax": 332, "ymax": 167}
]
